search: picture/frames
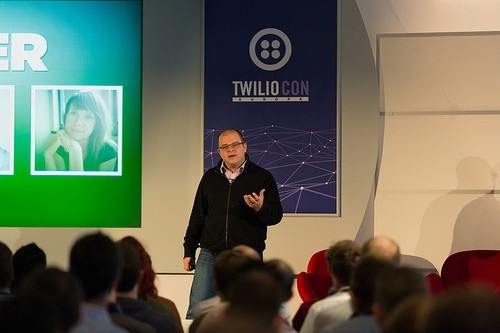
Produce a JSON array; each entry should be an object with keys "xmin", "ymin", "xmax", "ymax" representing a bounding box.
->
[{"xmin": 201, "ymin": 0, "xmax": 342, "ymax": 218}]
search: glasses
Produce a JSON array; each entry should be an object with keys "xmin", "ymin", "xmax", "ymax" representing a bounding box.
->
[{"xmin": 218, "ymin": 141, "xmax": 243, "ymax": 150}]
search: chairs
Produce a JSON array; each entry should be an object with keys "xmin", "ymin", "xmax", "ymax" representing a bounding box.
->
[
  {"xmin": 297, "ymin": 250, "xmax": 334, "ymax": 303},
  {"xmin": 424, "ymin": 250, "xmax": 500, "ymax": 310}
]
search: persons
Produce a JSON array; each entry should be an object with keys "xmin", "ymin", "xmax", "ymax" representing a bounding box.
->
[
  {"xmin": 182, "ymin": 128, "xmax": 284, "ymax": 319},
  {"xmin": 0, "ymin": 228, "xmax": 500, "ymax": 333},
  {"xmin": 42, "ymin": 90, "xmax": 119, "ymax": 172}
]
